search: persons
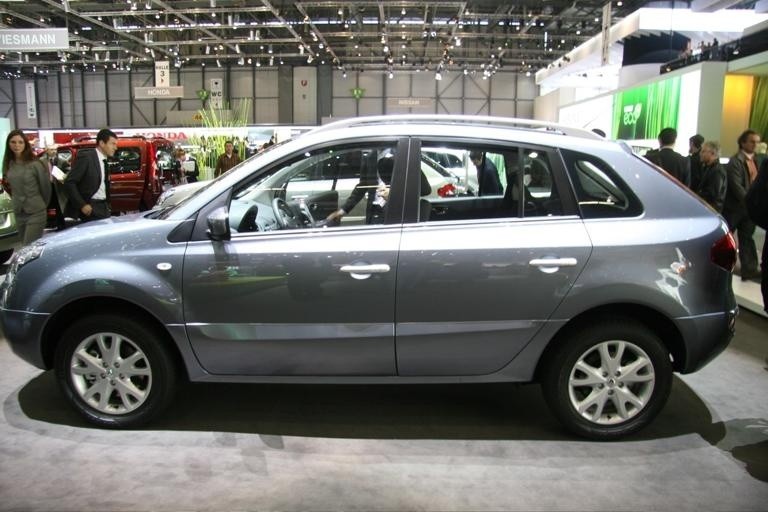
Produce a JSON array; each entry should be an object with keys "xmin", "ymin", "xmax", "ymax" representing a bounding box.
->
[
  {"xmin": 646, "ymin": 128, "xmax": 767, "ymax": 314},
  {"xmin": 214, "ymin": 141, "xmax": 241, "ymax": 178},
  {"xmin": 173, "ymin": 149, "xmax": 200, "ymax": 181},
  {"xmin": 470, "ymin": 150, "xmax": 504, "ymax": 196},
  {"xmin": 64, "ymin": 128, "xmax": 118, "ymax": 221},
  {"xmin": 269, "ymin": 136, "xmax": 275, "ymax": 145},
  {"xmin": 39, "ymin": 145, "xmax": 70, "ymax": 230},
  {"xmin": 327, "ymin": 146, "xmax": 395, "ymax": 223},
  {"xmin": 1, "ymin": 129, "xmax": 52, "ymax": 247}
]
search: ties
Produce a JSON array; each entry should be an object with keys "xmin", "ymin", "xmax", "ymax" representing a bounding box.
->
[{"xmin": 103, "ymin": 159, "xmax": 110, "ymax": 204}]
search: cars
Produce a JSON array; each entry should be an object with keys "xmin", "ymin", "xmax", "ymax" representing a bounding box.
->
[
  {"xmin": 421, "ymin": 148, "xmax": 484, "ymax": 192},
  {"xmin": 53, "ymin": 135, "xmax": 182, "ymax": 216},
  {"xmin": 149, "ymin": 150, "xmax": 481, "ymax": 224},
  {"xmin": 0, "ymin": 113, "xmax": 739, "ymax": 440},
  {"xmin": 0, "ymin": 189, "xmax": 22, "ymax": 268}
]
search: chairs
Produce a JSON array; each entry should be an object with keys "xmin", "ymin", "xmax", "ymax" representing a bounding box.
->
[{"xmin": 373, "ymin": 156, "xmax": 433, "ymax": 225}]
